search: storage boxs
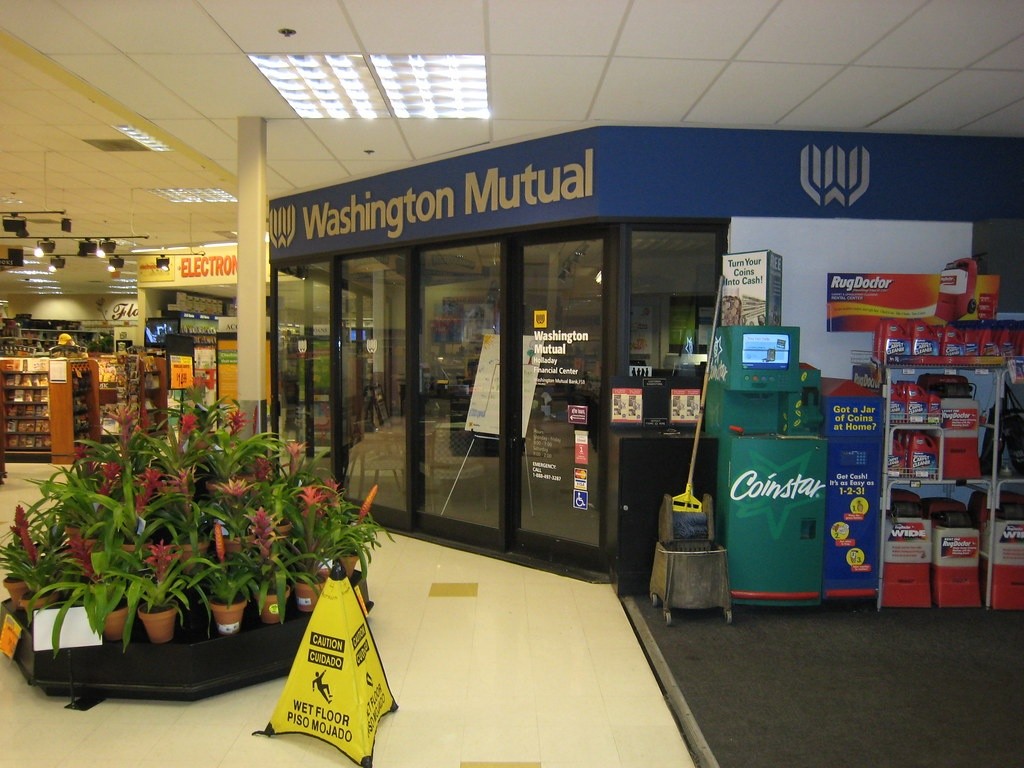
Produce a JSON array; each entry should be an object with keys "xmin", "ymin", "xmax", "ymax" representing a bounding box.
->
[
  {"xmin": 167, "ymin": 291, "xmax": 223, "ymax": 316},
  {"xmin": 721, "ymin": 248, "xmax": 783, "ymax": 327}
]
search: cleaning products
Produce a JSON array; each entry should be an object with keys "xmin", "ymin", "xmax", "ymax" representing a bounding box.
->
[{"xmin": 874, "ymin": 315, "xmax": 1024, "ymax": 482}]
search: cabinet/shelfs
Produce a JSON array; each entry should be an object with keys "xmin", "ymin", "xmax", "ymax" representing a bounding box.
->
[
  {"xmin": 19, "ymin": 328, "xmax": 98, "ymax": 351},
  {"xmin": 0, "ymin": 355, "xmax": 51, "ymax": 463},
  {"xmin": 48, "ymin": 357, "xmax": 101, "ymax": 465},
  {"xmin": 139, "ymin": 356, "xmax": 168, "ymax": 440},
  {"xmin": 866, "ymin": 354, "xmax": 1024, "ymax": 617},
  {"xmin": 95, "ymin": 357, "xmax": 121, "ymax": 444}
]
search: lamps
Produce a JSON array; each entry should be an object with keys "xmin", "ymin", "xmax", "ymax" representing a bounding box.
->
[
  {"xmin": 77, "ymin": 241, "xmax": 97, "ymax": 257},
  {"xmin": 156, "ymin": 257, "xmax": 170, "ymax": 271},
  {"xmin": 7, "ymin": 248, "xmax": 24, "ymax": 267},
  {"xmin": 48, "ymin": 257, "xmax": 66, "ymax": 272},
  {"xmin": 107, "ymin": 257, "xmax": 124, "ymax": 273},
  {"xmin": 96, "ymin": 242, "xmax": 116, "ymax": 258},
  {"xmin": 2, "ymin": 216, "xmax": 30, "ymax": 238},
  {"xmin": 557, "ymin": 244, "xmax": 590, "ymax": 284},
  {"xmin": 34, "ymin": 240, "xmax": 56, "ymax": 258}
]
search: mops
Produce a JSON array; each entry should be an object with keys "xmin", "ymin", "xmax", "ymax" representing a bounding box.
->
[{"xmin": 671, "ymin": 274, "xmax": 726, "ymax": 539}]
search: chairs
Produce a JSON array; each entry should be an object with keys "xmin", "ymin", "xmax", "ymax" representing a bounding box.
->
[{"xmin": 356, "ymin": 424, "xmax": 490, "ymax": 512}]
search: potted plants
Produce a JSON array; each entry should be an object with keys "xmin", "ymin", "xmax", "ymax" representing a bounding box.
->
[{"xmin": 0, "ymin": 377, "xmax": 395, "ymax": 643}]
